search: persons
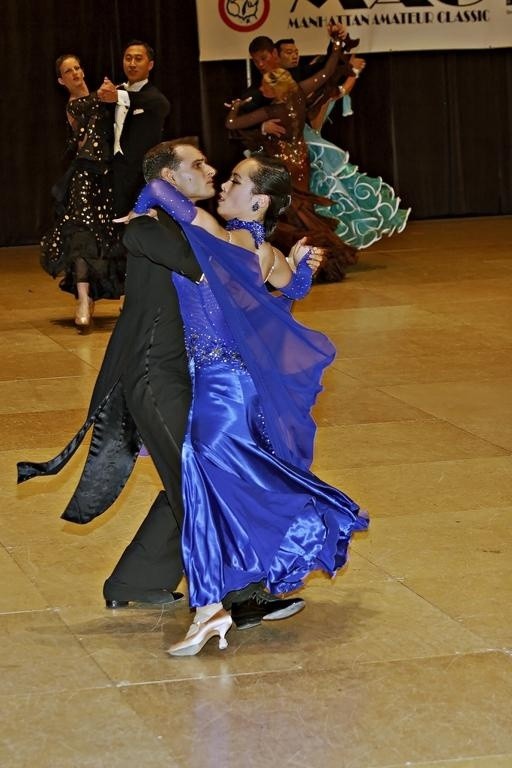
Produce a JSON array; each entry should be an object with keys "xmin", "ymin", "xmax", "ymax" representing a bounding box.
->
[
  {"xmin": 37, "ymin": 56, "xmax": 126, "ymax": 329},
  {"xmin": 110, "ymin": 154, "xmax": 369, "ymax": 655},
  {"xmin": 14, "ymin": 135, "xmax": 323, "ymax": 630},
  {"xmin": 222, "ymin": 21, "xmax": 413, "ymax": 290},
  {"xmin": 98, "ymin": 42, "xmax": 174, "ymax": 218}
]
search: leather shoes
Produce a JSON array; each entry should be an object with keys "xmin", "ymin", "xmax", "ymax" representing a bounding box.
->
[
  {"xmin": 230, "ymin": 587, "xmax": 306, "ymax": 630},
  {"xmin": 106, "ymin": 588, "xmax": 185, "ymax": 609},
  {"xmin": 75, "ymin": 299, "xmax": 94, "ymax": 327}
]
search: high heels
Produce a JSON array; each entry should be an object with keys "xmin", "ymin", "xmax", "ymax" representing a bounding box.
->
[{"xmin": 168, "ymin": 602, "xmax": 233, "ymax": 656}]
